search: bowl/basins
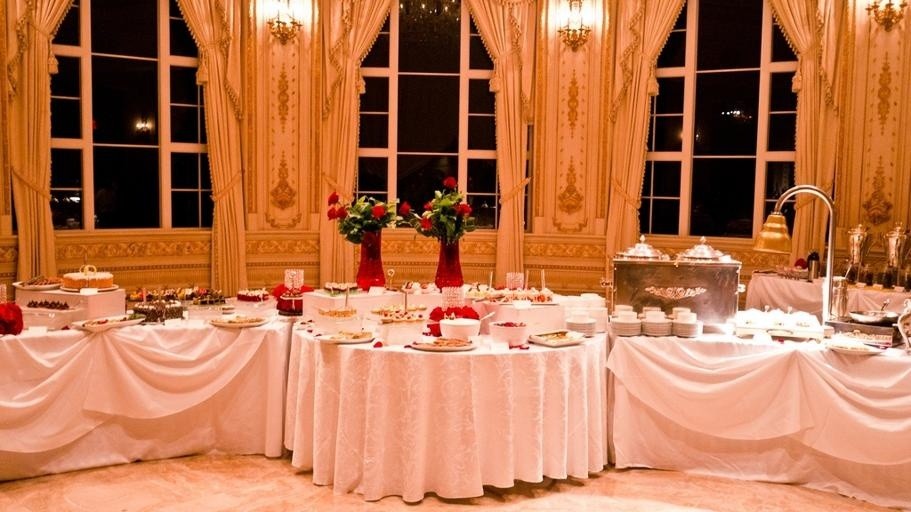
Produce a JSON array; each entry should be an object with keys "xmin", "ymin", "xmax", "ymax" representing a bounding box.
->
[
  {"xmin": 402, "ymin": 284, "xmax": 439, "ymax": 295},
  {"xmin": 613, "ymin": 305, "xmax": 698, "ymax": 321},
  {"xmin": 489, "ymin": 322, "xmax": 531, "ymax": 345},
  {"xmin": 440, "ymin": 318, "xmax": 482, "ymax": 338}
]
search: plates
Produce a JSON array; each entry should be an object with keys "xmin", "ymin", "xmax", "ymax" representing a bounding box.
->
[
  {"xmin": 477, "ymin": 294, "xmax": 557, "ymax": 306},
  {"xmin": 567, "ymin": 319, "xmax": 707, "ymax": 338},
  {"xmin": 410, "ymin": 335, "xmax": 479, "ymax": 351},
  {"xmin": 825, "ymin": 342, "xmax": 880, "ymax": 356},
  {"xmin": 10, "ymin": 280, "xmax": 183, "ymax": 333},
  {"xmin": 315, "ymin": 329, "xmax": 376, "ymax": 344},
  {"xmin": 209, "ymin": 314, "xmax": 267, "ymax": 328}
]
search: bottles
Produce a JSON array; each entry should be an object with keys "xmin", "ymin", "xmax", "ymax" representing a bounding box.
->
[
  {"xmin": 808, "ymin": 251, "xmax": 820, "ymax": 282},
  {"xmin": 845, "ymin": 223, "xmax": 911, "ymax": 293}
]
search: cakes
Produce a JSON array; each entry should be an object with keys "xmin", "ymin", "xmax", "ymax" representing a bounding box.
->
[{"xmin": 63, "ymin": 265, "xmax": 358, "ymax": 324}]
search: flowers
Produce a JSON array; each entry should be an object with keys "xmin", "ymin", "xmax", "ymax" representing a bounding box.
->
[
  {"xmin": 318, "ymin": 168, "xmax": 404, "ymax": 248},
  {"xmin": 391, "ymin": 173, "xmax": 479, "ymax": 248}
]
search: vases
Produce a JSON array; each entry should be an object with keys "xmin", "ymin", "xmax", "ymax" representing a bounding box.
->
[
  {"xmin": 353, "ymin": 227, "xmax": 388, "ymax": 293},
  {"xmin": 430, "ymin": 233, "xmax": 466, "ymax": 292}
]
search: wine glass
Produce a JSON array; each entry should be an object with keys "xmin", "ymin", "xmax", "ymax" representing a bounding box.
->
[
  {"xmin": 279, "ymin": 266, "xmax": 308, "ymax": 315},
  {"xmin": 507, "ymin": 271, "xmax": 525, "ymax": 299}
]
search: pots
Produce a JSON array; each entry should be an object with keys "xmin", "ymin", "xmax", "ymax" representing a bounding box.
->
[{"xmin": 847, "ymin": 298, "xmax": 901, "ymax": 325}]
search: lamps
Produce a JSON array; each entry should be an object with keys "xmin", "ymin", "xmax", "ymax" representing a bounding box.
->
[
  {"xmin": 554, "ymin": 0, "xmax": 593, "ymax": 56},
  {"xmin": 261, "ymin": 0, "xmax": 307, "ymax": 45},
  {"xmin": 865, "ymin": 0, "xmax": 910, "ymax": 33},
  {"xmin": 749, "ymin": 182, "xmax": 838, "ymax": 329}
]
